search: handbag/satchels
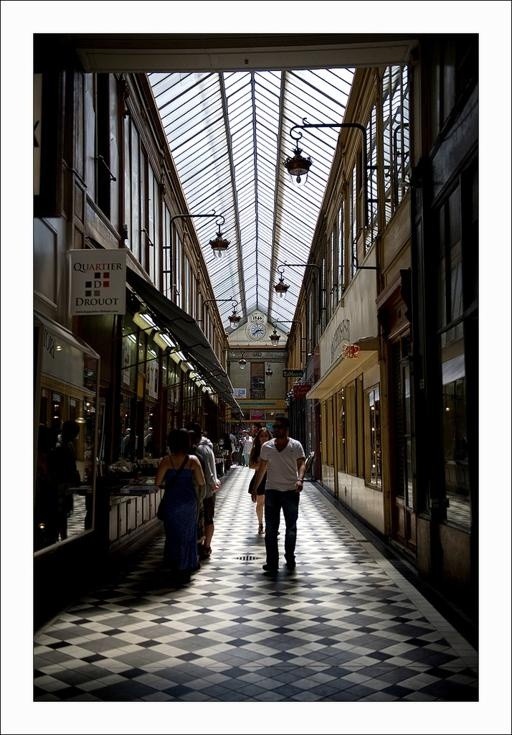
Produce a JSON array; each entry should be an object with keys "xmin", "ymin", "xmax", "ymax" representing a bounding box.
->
[{"xmin": 157, "ymin": 496, "xmax": 164, "ymax": 521}]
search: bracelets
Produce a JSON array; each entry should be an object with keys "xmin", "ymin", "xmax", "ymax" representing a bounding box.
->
[{"xmin": 297, "ymin": 478, "xmax": 304, "ymax": 482}]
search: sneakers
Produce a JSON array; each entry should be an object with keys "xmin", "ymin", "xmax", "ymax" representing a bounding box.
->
[
  {"xmin": 285, "ymin": 562, "xmax": 296, "ymax": 572},
  {"xmin": 262, "ymin": 560, "xmax": 279, "ymax": 572},
  {"xmin": 202, "ymin": 547, "xmax": 212, "ymax": 557}
]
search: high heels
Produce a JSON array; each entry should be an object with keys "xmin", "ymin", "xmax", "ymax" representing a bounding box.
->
[{"xmin": 258, "ymin": 524, "xmax": 264, "ymax": 535}]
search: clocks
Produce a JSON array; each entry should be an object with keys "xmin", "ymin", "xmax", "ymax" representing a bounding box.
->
[{"xmin": 249, "ymin": 322, "xmax": 266, "ymax": 339}]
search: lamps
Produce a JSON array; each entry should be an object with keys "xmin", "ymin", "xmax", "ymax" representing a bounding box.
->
[
  {"xmin": 171, "ymin": 208, "xmax": 241, "ymax": 329},
  {"xmin": 268, "ymin": 122, "xmax": 367, "ymax": 363}
]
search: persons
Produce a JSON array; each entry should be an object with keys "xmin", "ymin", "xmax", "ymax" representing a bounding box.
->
[
  {"xmin": 251, "ymin": 415, "xmax": 306, "ymax": 571},
  {"xmin": 250, "ymin": 427, "xmax": 282, "ymax": 536},
  {"xmin": 223, "ymin": 428, "xmax": 256, "ymax": 468},
  {"xmin": 36, "ymin": 410, "xmax": 162, "ymax": 547},
  {"xmin": 151, "ymin": 418, "xmax": 222, "ymax": 585}
]
